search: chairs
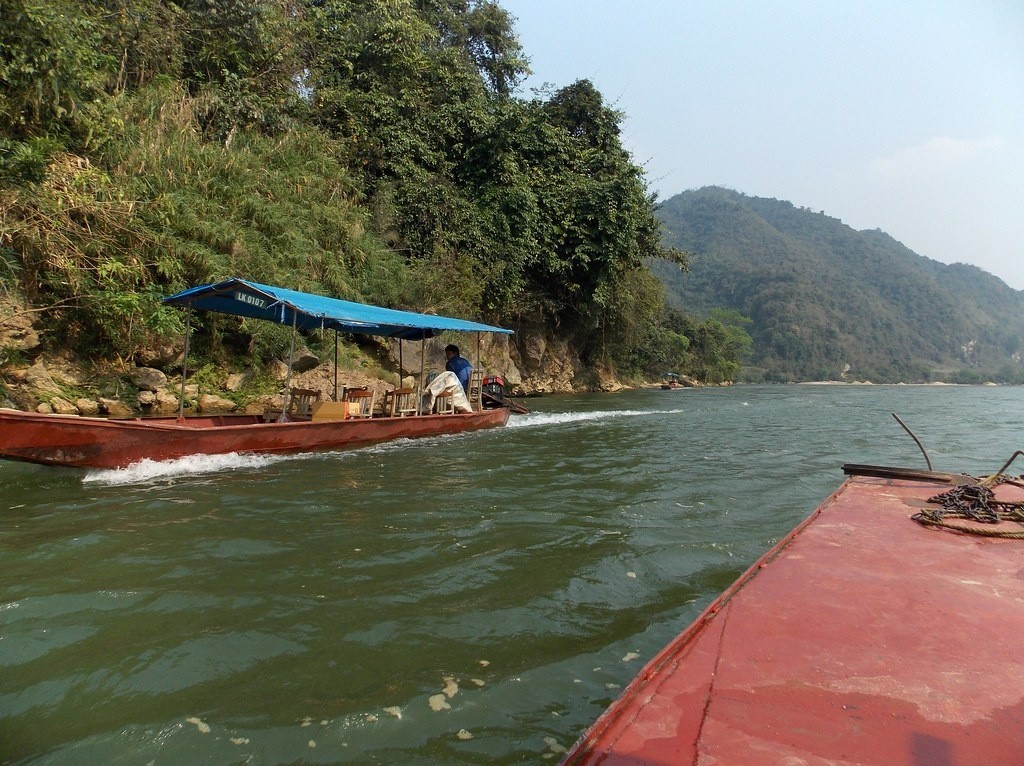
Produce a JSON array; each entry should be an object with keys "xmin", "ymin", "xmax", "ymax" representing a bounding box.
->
[
  {"xmin": 381, "ymin": 369, "xmax": 484, "ymax": 417},
  {"xmin": 265, "ymin": 385, "xmax": 375, "ymax": 424}
]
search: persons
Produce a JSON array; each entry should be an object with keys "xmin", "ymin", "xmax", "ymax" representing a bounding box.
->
[
  {"xmin": 669, "ymin": 376, "xmax": 676, "ymax": 384},
  {"xmin": 445, "ymin": 344, "xmax": 472, "ymax": 394}
]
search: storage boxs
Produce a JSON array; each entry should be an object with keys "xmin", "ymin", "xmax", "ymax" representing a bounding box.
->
[{"xmin": 311, "ymin": 402, "xmax": 359, "ymax": 421}]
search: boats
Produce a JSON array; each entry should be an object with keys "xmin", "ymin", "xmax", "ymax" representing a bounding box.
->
[
  {"xmin": 661, "ymin": 371, "xmax": 684, "ymax": 390},
  {"xmin": 0, "ymin": 273, "xmax": 532, "ymax": 471}
]
